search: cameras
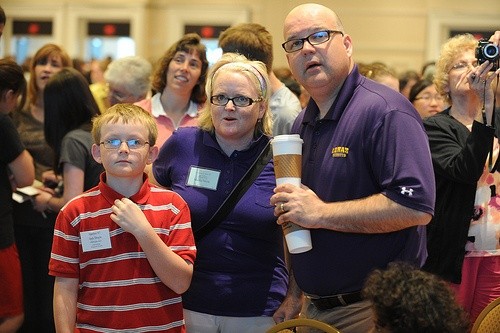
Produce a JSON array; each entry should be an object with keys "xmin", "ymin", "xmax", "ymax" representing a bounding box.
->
[{"xmin": 475, "ymin": 40, "xmax": 500, "ymax": 71}]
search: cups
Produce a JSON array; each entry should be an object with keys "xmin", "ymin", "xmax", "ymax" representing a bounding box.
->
[
  {"xmin": 275, "ymin": 203, "xmax": 312, "ymax": 254},
  {"xmin": 270, "ymin": 134, "xmax": 305, "ymax": 188}
]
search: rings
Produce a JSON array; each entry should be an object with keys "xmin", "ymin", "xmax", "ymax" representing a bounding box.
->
[
  {"xmin": 281, "ymin": 204, "xmax": 285, "ymax": 212},
  {"xmin": 471, "ymin": 73, "xmax": 476, "ymax": 80},
  {"xmin": 480, "ymin": 80, "xmax": 486, "ymax": 84}
]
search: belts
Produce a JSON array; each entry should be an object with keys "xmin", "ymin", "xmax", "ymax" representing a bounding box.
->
[{"xmin": 308, "ymin": 294, "xmax": 367, "ymax": 312}]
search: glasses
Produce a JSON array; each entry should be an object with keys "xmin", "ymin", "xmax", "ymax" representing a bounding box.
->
[
  {"xmin": 99, "ymin": 136, "xmax": 150, "ymax": 149},
  {"xmin": 447, "ymin": 62, "xmax": 478, "ymax": 74},
  {"xmin": 281, "ymin": 30, "xmax": 344, "ymax": 54},
  {"xmin": 414, "ymin": 96, "xmax": 446, "ymax": 103},
  {"xmin": 209, "ymin": 94, "xmax": 262, "ymax": 109}
]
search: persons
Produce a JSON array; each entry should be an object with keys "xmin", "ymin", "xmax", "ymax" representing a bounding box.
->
[
  {"xmin": 0, "ymin": 6, "xmax": 450, "ymax": 333},
  {"xmin": 364, "ymin": 261, "xmax": 469, "ymax": 333},
  {"xmin": 48, "ymin": 104, "xmax": 197, "ymax": 333},
  {"xmin": 271, "ymin": 4, "xmax": 435, "ymax": 333},
  {"xmin": 150, "ymin": 54, "xmax": 289, "ymax": 333},
  {"xmin": 423, "ymin": 32, "xmax": 500, "ymax": 333}
]
